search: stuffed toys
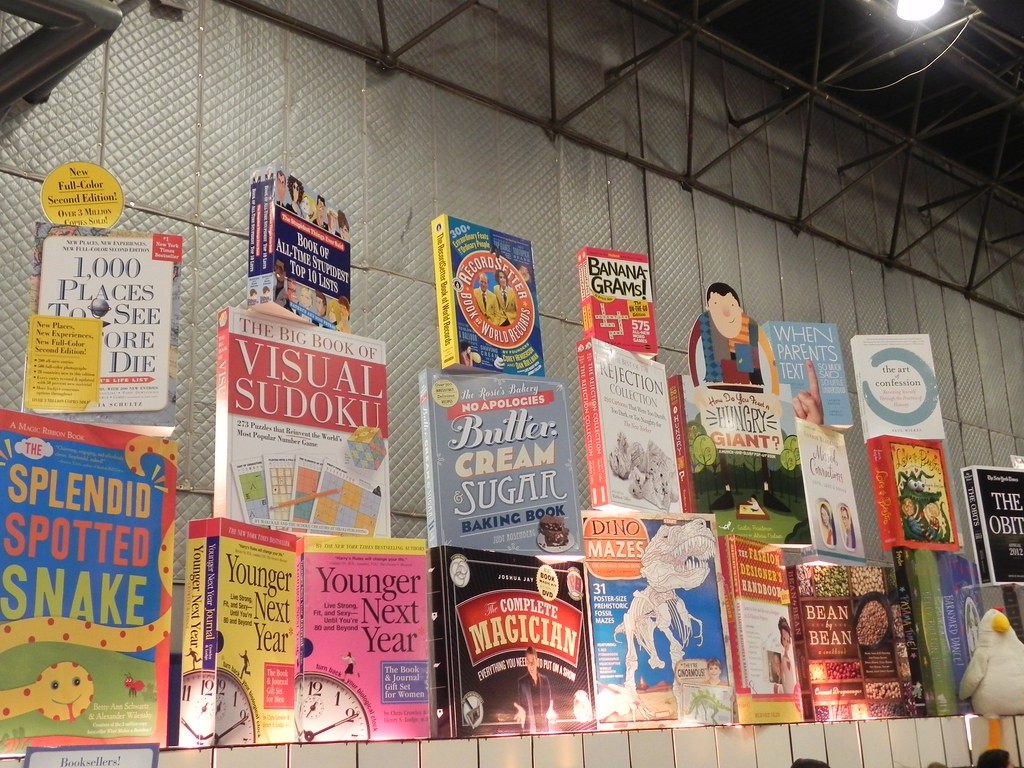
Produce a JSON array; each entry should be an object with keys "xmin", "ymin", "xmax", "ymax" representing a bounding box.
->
[{"xmin": 957, "ymin": 606, "xmax": 1024, "ymax": 767}]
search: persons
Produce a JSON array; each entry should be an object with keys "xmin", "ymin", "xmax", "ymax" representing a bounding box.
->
[
  {"xmin": 214, "ymin": 307, "xmax": 391, "ymax": 539},
  {"xmin": 514, "ymin": 645, "xmax": 551, "ymax": 735}
]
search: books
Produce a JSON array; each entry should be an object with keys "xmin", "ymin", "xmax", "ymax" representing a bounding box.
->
[
  {"xmin": 669, "ymin": 282, "xmax": 866, "ymax": 565},
  {"xmin": 418, "ymin": 367, "xmax": 585, "ymax": 561},
  {"xmin": 22, "ymin": 221, "xmax": 185, "ymax": 434},
  {"xmin": 0, "ymin": 409, "xmax": 181, "ymax": 751},
  {"xmin": 576, "ymin": 246, "xmax": 658, "ymax": 360},
  {"xmin": 850, "ymin": 334, "xmax": 946, "ymax": 443},
  {"xmin": 867, "ymin": 434, "xmax": 958, "ymax": 552},
  {"xmin": 962, "ymin": 465, "xmax": 1023, "ymax": 584},
  {"xmin": 431, "ymin": 214, "xmax": 545, "ymax": 375},
  {"xmin": 577, "ymin": 336, "xmax": 683, "ymax": 514},
  {"xmin": 247, "ymin": 165, "xmax": 352, "ymax": 332},
  {"xmin": 181, "ymin": 516, "xmax": 982, "ymax": 748}
]
